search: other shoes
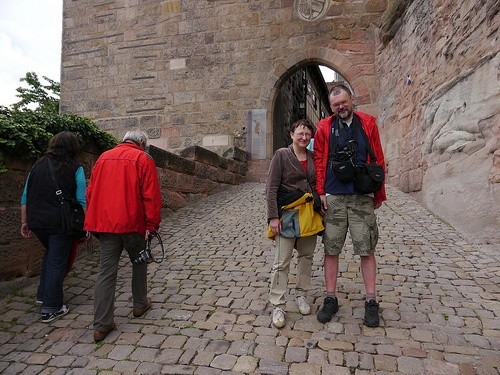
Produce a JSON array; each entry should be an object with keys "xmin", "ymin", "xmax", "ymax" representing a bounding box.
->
[
  {"xmin": 133, "ymin": 296, "xmax": 151, "ymax": 317},
  {"xmin": 94, "ymin": 320, "xmax": 115, "ymax": 342}
]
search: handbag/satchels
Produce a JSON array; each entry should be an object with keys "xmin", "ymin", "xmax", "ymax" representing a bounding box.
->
[
  {"xmin": 354, "ymin": 164, "xmax": 384, "ymax": 195},
  {"xmin": 56, "ymin": 189, "xmax": 87, "ymax": 242}
]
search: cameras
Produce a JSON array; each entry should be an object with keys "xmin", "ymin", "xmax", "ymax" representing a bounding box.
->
[{"xmin": 134, "ymin": 248, "xmax": 154, "ymax": 265}]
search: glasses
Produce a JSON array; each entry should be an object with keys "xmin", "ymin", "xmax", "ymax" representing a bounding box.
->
[{"xmin": 291, "ymin": 131, "xmax": 312, "ymax": 139}]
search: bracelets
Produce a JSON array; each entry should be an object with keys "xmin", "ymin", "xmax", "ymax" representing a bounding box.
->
[{"xmin": 21, "ymin": 221, "xmax": 27, "ymax": 224}]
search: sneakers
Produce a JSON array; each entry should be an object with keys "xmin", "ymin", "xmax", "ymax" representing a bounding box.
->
[
  {"xmin": 363, "ymin": 299, "xmax": 379, "ymax": 327},
  {"xmin": 36, "ymin": 296, "xmax": 43, "ymax": 304},
  {"xmin": 295, "ymin": 296, "xmax": 310, "ymax": 314},
  {"xmin": 41, "ymin": 305, "xmax": 70, "ymax": 322},
  {"xmin": 317, "ymin": 297, "xmax": 339, "ymax": 324},
  {"xmin": 272, "ymin": 306, "xmax": 286, "ymax": 328}
]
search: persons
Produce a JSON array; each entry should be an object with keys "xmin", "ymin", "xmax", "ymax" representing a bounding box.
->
[
  {"xmin": 21, "ymin": 132, "xmax": 87, "ymax": 322},
  {"xmin": 83, "ymin": 129, "xmax": 162, "ymax": 342},
  {"xmin": 313, "ymin": 84, "xmax": 387, "ymax": 328},
  {"xmin": 265, "ymin": 119, "xmax": 316, "ymax": 328}
]
